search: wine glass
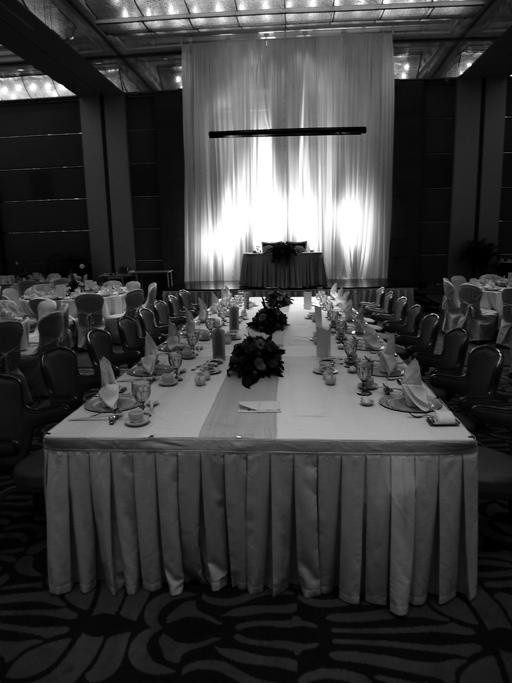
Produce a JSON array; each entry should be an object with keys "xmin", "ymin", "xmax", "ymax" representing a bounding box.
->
[
  {"xmin": 314, "ymin": 284, "xmax": 373, "ymax": 396},
  {"xmin": 0, "ymin": 270, "xmax": 49, "ymax": 284},
  {"xmin": 124, "ymin": 286, "xmax": 257, "ymax": 427},
  {"xmin": 21, "ymin": 278, "xmax": 124, "ymax": 301}
]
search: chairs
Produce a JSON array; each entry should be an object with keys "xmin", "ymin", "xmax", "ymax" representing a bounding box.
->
[
  {"xmin": 0, "ymin": 273, "xmax": 200, "ymax": 374},
  {"xmin": 0, "ymin": 374, "xmax": 71, "ymax": 476},
  {"xmin": 430, "ymin": 327, "xmax": 469, "ymax": 376},
  {"xmin": 359, "ymin": 273, "xmax": 512, "ymax": 345},
  {"xmin": 469, "ymin": 404, "xmax": 512, "ymax": 515},
  {"xmin": 430, "ymin": 345, "xmax": 504, "ymax": 409}
]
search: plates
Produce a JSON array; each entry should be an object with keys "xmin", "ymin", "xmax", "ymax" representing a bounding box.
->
[
  {"xmin": 82, "ymin": 293, "xmax": 239, "ymax": 414},
  {"xmin": 332, "ymin": 293, "xmax": 445, "ymax": 413},
  {"xmin": 471, "ymin": 276, "xmax": 512, "ymax": 291}
]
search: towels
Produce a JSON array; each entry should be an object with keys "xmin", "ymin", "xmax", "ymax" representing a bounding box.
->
[
  {"xmin": 100, "ymin": 285, "xmax": 231, "ymax": 410},
  {"xmin": 330, "ymin": 282, "xmax": 434, "ymax": 413}
]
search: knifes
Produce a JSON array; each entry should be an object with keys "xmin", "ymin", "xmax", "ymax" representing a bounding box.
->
[{"xmin": 67, "ymin": 416, "xmax": 108, "ymax": 423}]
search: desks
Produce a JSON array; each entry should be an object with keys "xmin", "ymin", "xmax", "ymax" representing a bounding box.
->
[{"xmin": 239, "ymin": 252, "xmax": 328, "ymax": 288}]
